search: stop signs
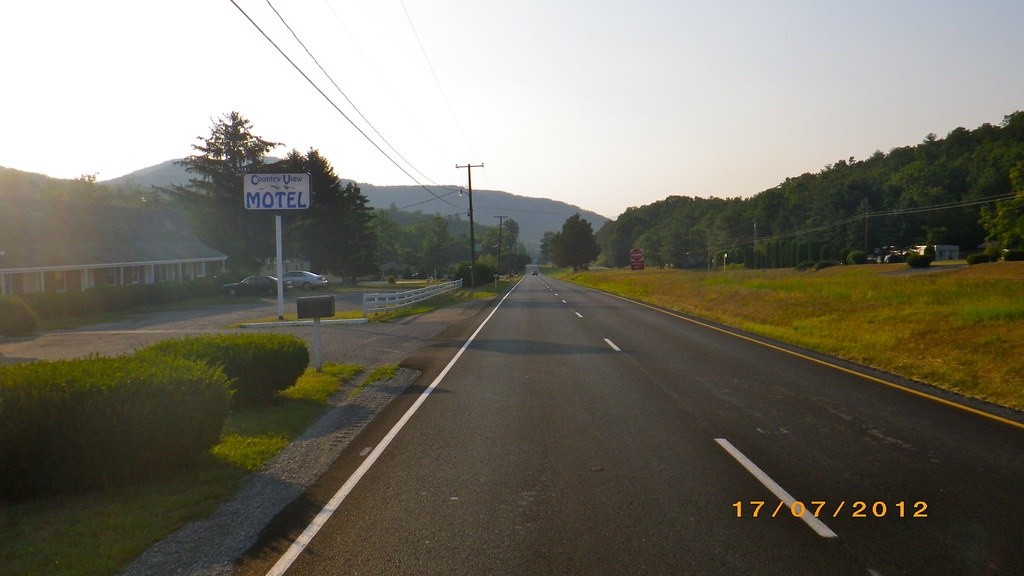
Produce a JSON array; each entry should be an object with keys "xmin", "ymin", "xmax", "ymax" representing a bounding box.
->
[{"xmin": 630, "ymin": 249, "xmax": 643, "ymax": 261}]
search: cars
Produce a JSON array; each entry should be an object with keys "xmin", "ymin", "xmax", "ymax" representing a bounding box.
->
[
  {"xmin": 284, "ymin": 270, "xmax": 328, "ymax": 290},
  {"xmin": 532, "ymin": 270, "xmax": 538, "ymax": 276},
  {"xmin": 219, "ymin": 275, "xmax": 293, "ymax": 296}
]
